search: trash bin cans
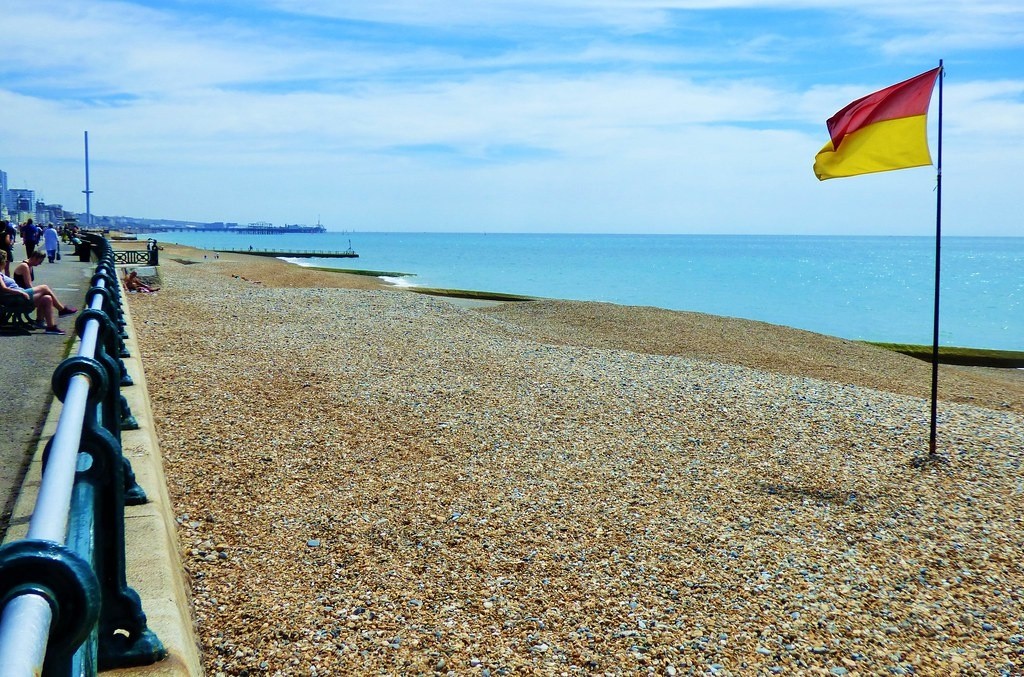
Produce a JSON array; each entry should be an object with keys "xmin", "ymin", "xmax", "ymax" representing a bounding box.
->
[{"xmin": 80, "ymin": 240, "xmax": 91, "ymax": 262}]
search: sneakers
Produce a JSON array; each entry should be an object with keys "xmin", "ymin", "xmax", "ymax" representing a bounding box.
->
[
  {"xmin": 45, "ymin": 325, "xmax": 66, "ymax": 335},
  {"xmin": 58, "ymin": 306, "xmax": 78, "ymax": 318}
]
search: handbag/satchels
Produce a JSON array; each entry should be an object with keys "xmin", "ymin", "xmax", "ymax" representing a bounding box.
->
[{"xmin": 56, "ymin": 253, "xmax": 60, "ymax": 260}]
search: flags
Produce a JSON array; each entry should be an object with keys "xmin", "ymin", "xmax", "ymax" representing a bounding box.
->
[{"xmin": 813, "ymin": 65, "xmax": 941, "ymax": 182}]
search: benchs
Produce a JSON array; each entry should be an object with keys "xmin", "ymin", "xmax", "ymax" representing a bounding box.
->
[
  {"xmin": 0, "ymin": 285, "xmax": 46, "ymax": 337},
  {"xmin": 65, "ymin": 231, "xmax": 91, "ymax": 262}
]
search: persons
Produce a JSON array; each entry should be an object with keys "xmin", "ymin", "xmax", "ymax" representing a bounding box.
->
[
  {"xmin": 13, "ymin": 250, "xmax": 48, "ymax": 328},
  {"xmin": 125, "ymin": 269, "xmax": 149, "ymax": 290},
  {"xmin": 57, "ymin": 223, "xmax": 78, "ymax": 244},
  {"xmin": 43, "ymin": 222, "xmax": 59, "ymax": 264},
  {"xmin": 18, "ymin": 218, "xmax": 43, "ymax": 259},
  {"xmin": 0, "ymin": 219, "xmax": 17, "ymax": 277},
  {"xmin": 0, "ymin": 249, "xmax": 78, "ymax": 334}
]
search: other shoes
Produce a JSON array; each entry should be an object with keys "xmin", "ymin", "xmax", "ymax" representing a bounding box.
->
[
  {"xmin": 33, "ymin": 319, "xmax": 47, "ymax": 329},
  {"xmin": 49, "ymin": 257, "xmax": 54, "ymax": 263}
]
